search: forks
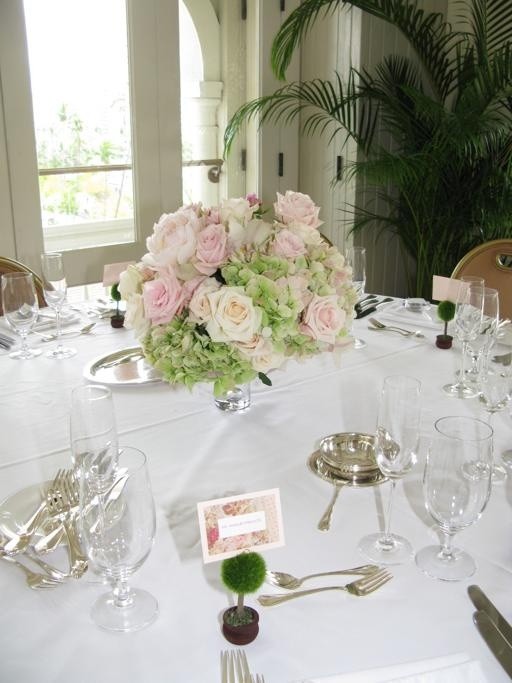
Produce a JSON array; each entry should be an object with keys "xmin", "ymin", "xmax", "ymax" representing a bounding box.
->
[
  {"xmin": 221, "ymin": 649, "xmax": 266, "ymax": 682},
  {"xmin": 258, "ymin": 568, "xmax": 394, "ymax": 607},
  {"xmin": 368, "ymin": 317, "xmax": 425, "ymax": 339},
  {"xmin": 0, "ymin": 442, "xmax": 130, "ymax": 588}
]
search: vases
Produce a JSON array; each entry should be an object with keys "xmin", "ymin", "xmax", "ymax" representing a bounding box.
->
[{"xmin": 212, "ymin": 373, "xmax": 252, "ymax": 412}]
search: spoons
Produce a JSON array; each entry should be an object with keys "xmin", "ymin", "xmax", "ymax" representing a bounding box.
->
[
  {"xmin": 262, "ymin": 564, "xmax": 379, "ymax": 590},
  {"xmin": 42, "ymin": 323, "xmax": 97, "ymax": 342},
  {"xmin": 368, "ymin": 326, "xmax": 422, "ymax": 338}
]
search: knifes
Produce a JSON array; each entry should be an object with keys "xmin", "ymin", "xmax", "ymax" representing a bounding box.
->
[
  {"xmin": 353, "ymin": 298, "xmax": 394, "ymax": 319},
  {"xmin": 467, "ymin": 584, "xmax": 512, "ymax": 682}
]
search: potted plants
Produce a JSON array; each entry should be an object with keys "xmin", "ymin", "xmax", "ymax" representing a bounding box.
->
[
  {"xmin": 109, "ymin": 282, "xmax": 125, "ymax": 328},
  {"xmin": 219, "ymin": 550, "xmax": 267, "ymax": 646},
  {"xmin": 436, "ymin": 296, "xmax": 454, "ymax": 349}
]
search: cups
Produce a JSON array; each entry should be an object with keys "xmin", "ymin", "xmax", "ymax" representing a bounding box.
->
[{"xmin": 405, "ymin": 297, "xmax": 431, "ymax": 312}]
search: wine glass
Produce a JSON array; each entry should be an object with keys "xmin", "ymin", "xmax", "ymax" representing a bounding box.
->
[
  {"xmin": 358, "ymin": 373, "xmax": 422, "ymax": 567},
  {"xmin": 415, "ymin": 415, "xmax": 494, "ymax": 583},
  {"xmin": 41, "ymin": 253, "xmax": 77, "ymax": 359},
  {"xmin": 78, "ymin": 446, "xmax": 160, "ymax": 633},
  {"xmin": 69, "ymin": 383, "xmax": 130, "ymax": 564},
  {"xmin": 1, "ymin": 272, "xmax": 44, "ymax": 360},
  {"xmin": 462, "ymin": 327, "xmax": 512, "ymax": 482},
  {"xmin": 344, "ymin": 246, "xmax": 367, "ymax": 350},
  {"xmin": 441, "ymin": 275, "xmax": 500, "ymax": 399}
]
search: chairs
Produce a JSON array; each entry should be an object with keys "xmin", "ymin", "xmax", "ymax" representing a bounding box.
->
[
  {"xmin": 448, "ymin": 238, "xmax": 510, "ymax": 323},
  {"xmin": 0, "ymin": 255, "xmax": 54, "ymax": 315}
]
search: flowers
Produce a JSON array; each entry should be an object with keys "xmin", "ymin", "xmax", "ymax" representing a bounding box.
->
[{"xmin": 120, "ymin": 196, "xmax": 365, "ymax": 401}]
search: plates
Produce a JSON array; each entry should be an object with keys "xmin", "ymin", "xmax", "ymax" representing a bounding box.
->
[
  {"xmin": 0, "ymin": 479, "xmax": 126, "ymax": 549},
  {"xmin": 83, "ymin": 344, "xmax": 164, "ymax": 386},
  {"xmin": 309, "ymin": 431, "xmax": 401, "ymax": 488}
]
